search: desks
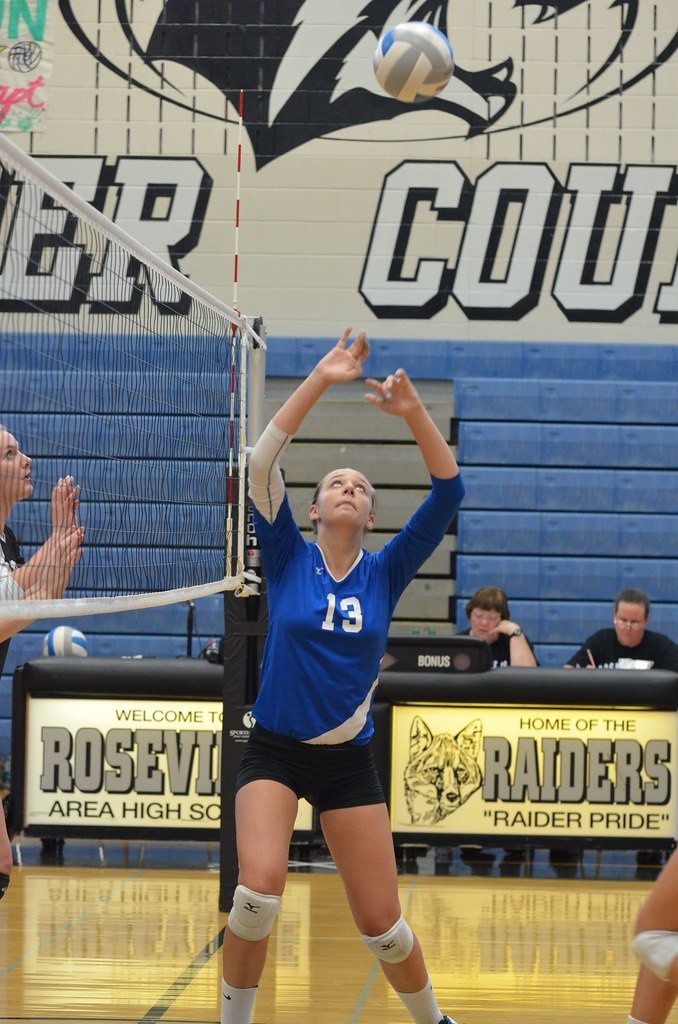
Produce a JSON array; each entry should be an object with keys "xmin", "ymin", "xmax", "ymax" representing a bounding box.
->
[{"xmin": 8, "ymin": 658, "xmax": 678, "ymax": 862}]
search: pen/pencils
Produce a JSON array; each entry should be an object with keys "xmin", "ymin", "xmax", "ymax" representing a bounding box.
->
[{"xmin": 587, "ymin": 650, "xmax": 597, "ymax": 669}]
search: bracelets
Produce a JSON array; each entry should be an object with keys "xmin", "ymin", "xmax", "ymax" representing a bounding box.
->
[{"xmin": 509, "ymin": 628, "xmax": 523, "ymax": 637}]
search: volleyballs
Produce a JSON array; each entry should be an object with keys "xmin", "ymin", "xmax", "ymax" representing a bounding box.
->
[
  {"xmin": 373, "ymin": 21, "xmax": 454, "ymax": 105},
  {"xmin": 43, "ymin": 623, "xmax": 89, "ymax": 659}
]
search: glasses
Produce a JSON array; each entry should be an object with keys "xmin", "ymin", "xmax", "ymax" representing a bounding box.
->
[{"xmin": 613, "ymin": 617, "xmax": 646, "ymax": 631}]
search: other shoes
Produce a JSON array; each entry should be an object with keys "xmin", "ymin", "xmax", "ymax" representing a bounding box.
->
[{"xmin": 438, "ymin": 1016, "xmax": 457, "ymax": 1024}]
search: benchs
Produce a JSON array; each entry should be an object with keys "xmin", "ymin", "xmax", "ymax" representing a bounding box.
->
[{"xmin": 1, "ymin": 331, "xmax": 678, "ymax": 668}]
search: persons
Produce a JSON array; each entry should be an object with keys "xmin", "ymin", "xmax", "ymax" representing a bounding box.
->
[
  {"xmin": 626, "ymin": 847, "xmax": 678, "ymax": 1024},
  {"xmin": 0, "ymin": 425, "xmax": 86, "ymax": 900},
  {"xmin": 456, "ymin": 586, "xmax": 539, "ymax": 669},
  {"xmin": 220, "ymin": 327, "xmax": 464, "ymax": 1024},
  {"xmin": 562, "ymin": 588, "xmax": 678, "ymax": 673}
]
